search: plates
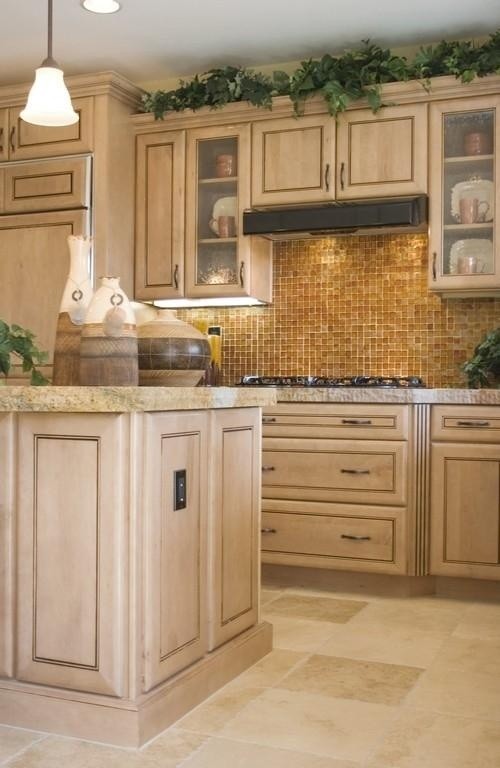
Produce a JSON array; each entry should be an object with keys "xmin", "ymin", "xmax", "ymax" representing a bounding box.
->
[
  {"xmin": 213, "ymin": 197, "xmax": 236, "ymax": 239},
  {"xmin": 447, "ymin": 239, "xmax": 495, "ymax": 275},
  {"xmin": 450, "ymin": 180, "xmax": 495, "ymax": 222}
]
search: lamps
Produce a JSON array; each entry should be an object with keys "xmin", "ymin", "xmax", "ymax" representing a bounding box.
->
[{"xmin": 19, "ymin": 1, "xmax": 79, "ymax": 131}]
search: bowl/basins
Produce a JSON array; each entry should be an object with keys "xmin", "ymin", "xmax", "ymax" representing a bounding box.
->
[
  {"xmin": 212, "ymin": 155, "xmax": 236, "ymax": 178},
  {"xmin": 462, "ymin": 132, "xmax": 493, "ymax": 155}
]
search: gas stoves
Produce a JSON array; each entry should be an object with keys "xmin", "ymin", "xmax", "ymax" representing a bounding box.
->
[{"xmin": 239, "ymin": 374, "xmax": 426, "ymax": 389}]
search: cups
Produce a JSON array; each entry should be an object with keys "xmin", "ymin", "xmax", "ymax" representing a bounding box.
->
[
  {"xmin": 459, "ymin": 197, "xmax": 488, "ymax": 222},
  {"xmin": 459, "ymin": 256, "xmax": 484, "ymax": 274},
  {"xmin": 206, "ymin": 215, "xmax": 235, "ymax": 238}
]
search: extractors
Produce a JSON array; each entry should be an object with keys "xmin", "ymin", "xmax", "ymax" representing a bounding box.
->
[{"xmin": 242, "ymin": 201, "xmax": 428, "ymax": 243}]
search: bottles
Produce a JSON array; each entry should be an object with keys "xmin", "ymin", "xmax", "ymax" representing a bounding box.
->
[{"xmin": 205, "ymin": 326, "xmax": 221, "ymax": 387}]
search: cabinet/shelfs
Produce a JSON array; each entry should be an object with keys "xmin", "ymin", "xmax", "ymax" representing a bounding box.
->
[
  {"xmin": 2, "ymin": 412, "xmax": 131, "ymax": 750},
  {"xmin": 262, "ymin": 404, "xmax": 417, "ymax": 577},
  {"xmin": 135, "ymin": 127, "xmax": 188, "ymax": 295},
  {"xmin": 252, "ymin": 95, "xmax": 431, "ymax": 210},
  {"xmin": 188, "ymin": 127, "xmax": 274, "ymax": 305},
  {"xmin": 417, "ymin": 404, "xmax": 499, "ymax": 576},
  {"xmin": 429, "ymin": 87, "xmax": 500, "ymax": 285},
  {"xmin": 0, "ymin": 91, "xmax": 134, "ymax": 211},
  {"xmin": 132, "ymin": 408, "xmax": 288, "ymax": 748}
]
